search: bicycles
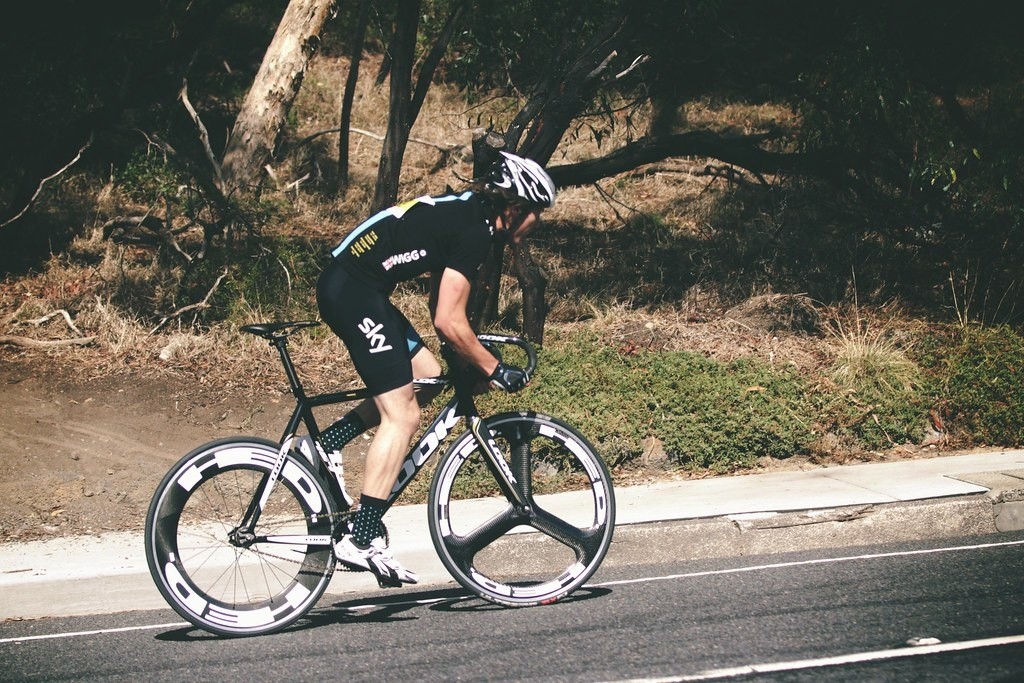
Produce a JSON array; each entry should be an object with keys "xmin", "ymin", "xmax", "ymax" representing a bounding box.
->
[{"xmin": 143, "ymin": 316, "xmax": 619, "ymax": 637}]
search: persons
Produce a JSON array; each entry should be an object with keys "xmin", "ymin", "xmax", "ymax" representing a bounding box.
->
[{"xmin": 296, "ymin": 150, "xmax": 556, "ymax": 586}]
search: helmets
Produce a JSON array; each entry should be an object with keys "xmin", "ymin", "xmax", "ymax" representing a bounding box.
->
[{"xmin": 478, "ymin": 151, "xmax": 555, "ymax": 212}]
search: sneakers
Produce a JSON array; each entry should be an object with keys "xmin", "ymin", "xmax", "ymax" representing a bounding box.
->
[
  {"xmin": 334, "ymin": 534, "xmax": 419, "ymax": 584},
  {"xmin": 294, "ymin": 434, "xmax": 354, "ymax": 508}
]
